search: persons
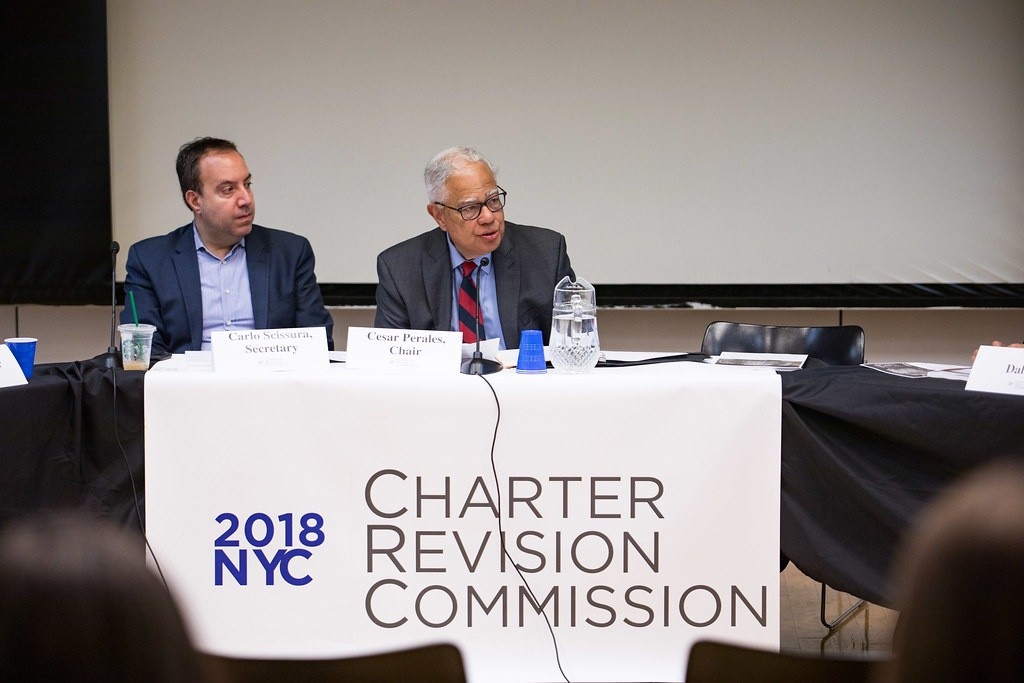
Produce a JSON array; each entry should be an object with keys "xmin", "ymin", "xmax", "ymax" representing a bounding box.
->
[
  {"xmin": 374, "ymin": 144, "xmax": 578, "ymax": 350},
  {"xmin": 120, "ymin": 136, "xmax": 336, "ymax": 370}
]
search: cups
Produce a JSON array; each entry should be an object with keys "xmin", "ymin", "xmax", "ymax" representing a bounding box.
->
[
  {"xmin": 516, "ymin": 330, "xmax": 547, "ymax": 374},
  {"xmin": 3, "ymin": 337, "xmax": 37, "ymax": 380},
  {"xmin": 117, "ymin": 323, "xmax": 157, "ymax": 370}
]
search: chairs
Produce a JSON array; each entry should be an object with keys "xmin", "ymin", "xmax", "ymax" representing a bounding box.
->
[{"xmin": 698, "ymin": 319, "xmax": 868, "ymax": 631}]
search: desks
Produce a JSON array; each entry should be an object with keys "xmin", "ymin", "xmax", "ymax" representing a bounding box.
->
[{"xmin": 0, "ymin": 359, "xmax": 1024, "ymax": 683}]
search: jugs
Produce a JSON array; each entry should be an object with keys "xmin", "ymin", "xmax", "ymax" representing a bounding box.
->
[{"xmin": 549, "ymin": 276, "xmax": 601, "ymax": 374}]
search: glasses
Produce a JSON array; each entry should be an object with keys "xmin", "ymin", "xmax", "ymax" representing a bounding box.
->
[{"xmin": 434, "ymin": 185, "xmax": 507, "ymax": 221}]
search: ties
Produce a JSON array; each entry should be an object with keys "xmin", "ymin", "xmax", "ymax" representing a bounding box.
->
[{"xmin": 459, "ymin": 261, "xmax": 486, "ymax": 343}]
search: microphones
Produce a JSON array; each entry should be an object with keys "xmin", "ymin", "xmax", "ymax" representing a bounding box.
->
[
  {"xmin": 89, "ymin": 241, "xmax": 124, "ymax": 371},
  {"xmin": 460, "ymin": 257, "xmax": 503, "ymax": 375}
]
